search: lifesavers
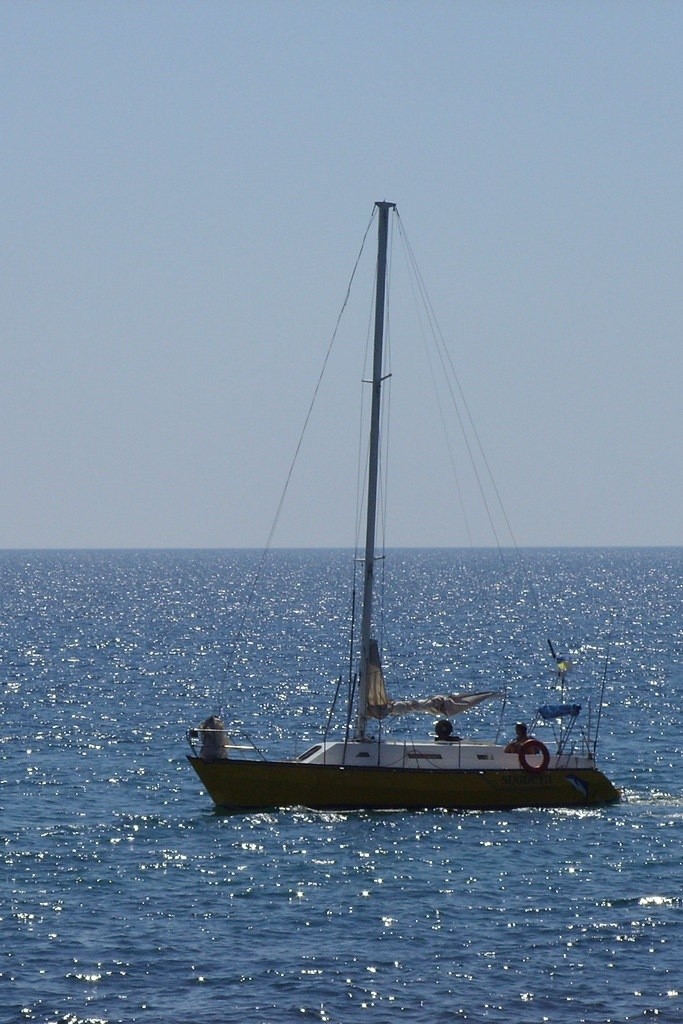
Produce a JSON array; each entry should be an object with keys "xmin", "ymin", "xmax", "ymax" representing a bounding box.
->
[
  {"xmin": 519, "ymin": 739, "xmax": 550, "ymax": 773},
  {"xmin": 435, "ymin": 719, "xmax": 453, "ymax": 740}
]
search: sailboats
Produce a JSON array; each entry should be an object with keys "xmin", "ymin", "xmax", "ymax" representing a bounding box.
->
[{"xmin": 184, "ymin": 200, "xmax": 626, "ymax": 809}]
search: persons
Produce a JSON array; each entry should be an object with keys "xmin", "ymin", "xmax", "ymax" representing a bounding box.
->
[{"xmin": 506, "ymin": 722, "xmax": 540, "ymax": 755}]
